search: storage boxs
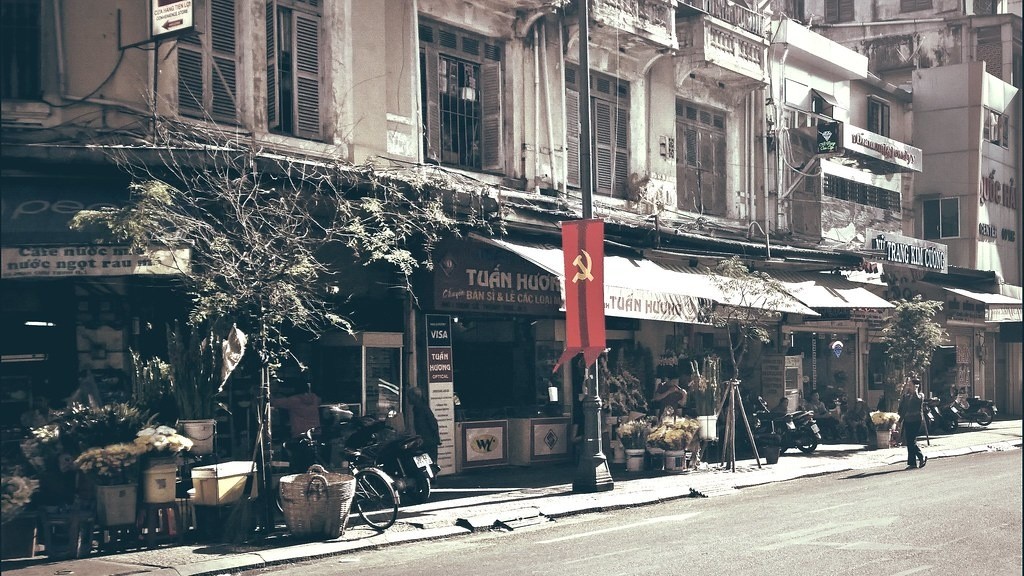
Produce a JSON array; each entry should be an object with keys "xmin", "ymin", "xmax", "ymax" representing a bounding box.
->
[{"xmin": 191, "ymin": 460, "xmax": 258, "ymax": 505}]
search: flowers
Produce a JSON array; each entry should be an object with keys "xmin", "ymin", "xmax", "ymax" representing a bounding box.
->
[
  {"xmin": 616, "ymin": 417, "xmax": 701, "ymax": 451},
  {"xmin": 0, "ymin": 402, "xmax": 194, "ymax": 525}
]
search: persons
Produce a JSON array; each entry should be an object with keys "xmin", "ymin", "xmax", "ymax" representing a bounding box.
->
[
  {"xmin": 407, "ymin": 387, "xmax": 440, "ymax": 489},
  {"xmin": 809, "ymin": 391, "xmax": 839, "ymax": 440},
  {"xmin": 272, "ymin": 382, "xmax": 322, "ymax": 473},
  {"xmin": 898, "ymin": 379, "xmax": 927, "ymax": 469},
  {"xmin": 653, "ymin": 373, "xmax": 687, "ymax": 420},
  {"xmin": 771, "ymin": 397, "xmax": 789, "ymax": 413}
]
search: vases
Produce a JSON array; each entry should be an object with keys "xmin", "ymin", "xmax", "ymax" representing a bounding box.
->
[{"xmin": 94, "ymin": 480, "xmax": 137, "ymax": 526}]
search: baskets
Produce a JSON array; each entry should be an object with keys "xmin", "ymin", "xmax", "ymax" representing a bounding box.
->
[
  {"xmin": 283, "ymin": 437, "xmax": 315, "ymax": 464},
  {"xmin": 279, "ymin": 465, "xmax": 356, "ymax": 539}
]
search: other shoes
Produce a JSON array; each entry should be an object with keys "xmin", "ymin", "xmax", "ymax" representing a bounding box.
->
[
  {"xmin": 906, "ymin": 466, "xmax": 916, "ymax": 469},
  {"xmin": 919, "ymin": 455, "xmax": 927, "ymax": 467}
]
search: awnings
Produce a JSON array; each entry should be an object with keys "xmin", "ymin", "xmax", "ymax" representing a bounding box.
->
[
  {"xmin": 914, "ymin": 278, "xmax": 1022, "ymax": 323},
  {"xmin": 468, "ymin": 233, "xmax": 897, "ymax": 326}
]
